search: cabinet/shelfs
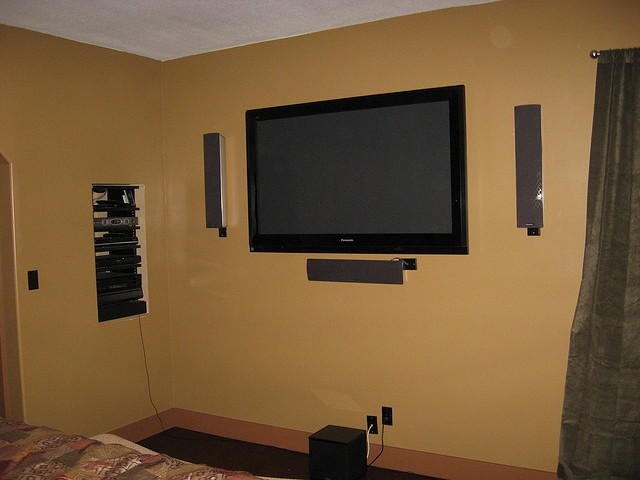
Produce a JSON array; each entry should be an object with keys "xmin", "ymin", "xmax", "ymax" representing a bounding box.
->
[{"xmin": 91, "ymin": 183, "xmax": 150, "ymax": 324}]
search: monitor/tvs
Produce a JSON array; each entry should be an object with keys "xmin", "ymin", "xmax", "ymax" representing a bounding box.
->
[{"xmin": 246, "ymin": 85, "xmax": 467, "ymax": 254}]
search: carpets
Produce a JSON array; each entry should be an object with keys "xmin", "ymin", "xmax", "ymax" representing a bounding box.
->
[{"xmin": 138, "ymin": 426, "xmax": 443, "ymax": 480}]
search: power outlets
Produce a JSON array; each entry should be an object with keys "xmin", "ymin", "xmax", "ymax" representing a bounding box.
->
[{"xmin": 382, "ymin": 406, "xmax": 392, "ymax": 425}]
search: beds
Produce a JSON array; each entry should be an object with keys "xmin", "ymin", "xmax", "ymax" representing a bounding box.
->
[{"xmin": 0, "ymin": 434, "xmax": 280, "ymax": 479}]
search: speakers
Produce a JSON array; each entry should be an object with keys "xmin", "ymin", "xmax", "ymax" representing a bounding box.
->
[
  {"xmin": 307, "ymin": 423, "xmax": 369, "ymax": 479},
  {"xmin": 203, "ymin": 133, "xmax": 228, "ymax": 228},
  {"xmin": 307, "ymin": 258, "xmax": 403, "ymax": 285},
  {"xmin": 514, "ymin": 104, "xmax": 543, "ymax": 228}
]
